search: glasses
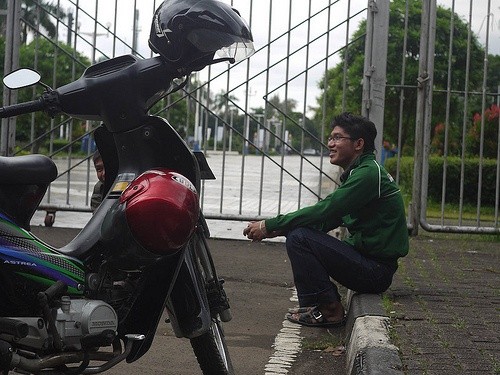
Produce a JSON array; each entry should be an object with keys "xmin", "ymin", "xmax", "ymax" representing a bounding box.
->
[{"xmin": 328, "ymin": 135, "xmax": 357, "ymax": 142}]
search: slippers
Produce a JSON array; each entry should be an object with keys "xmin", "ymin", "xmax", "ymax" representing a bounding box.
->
[
  {"xmin": 287, "ymin": 306, "xmax": 345, "ymax": 327},
  {"xmin": 288, "ymin": 307, "xmax": 313, "ymax": 314}
]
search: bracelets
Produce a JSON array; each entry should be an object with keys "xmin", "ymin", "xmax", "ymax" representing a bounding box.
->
[{"xmin": 259, "ymin": 220, "xmax": 267, "ymax": 237}]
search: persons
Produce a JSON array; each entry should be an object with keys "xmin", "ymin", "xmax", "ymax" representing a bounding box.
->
[
  {"xmin": 243, "ymin": 111, "xmax": 409, "ymax": 327},
  {"xmin": 381, "ymin": 140, "xmax": 398, "ymax": 163},
  {"xmin": 91, "ymin": 150, "xmax": 107, "ymax": 214}
]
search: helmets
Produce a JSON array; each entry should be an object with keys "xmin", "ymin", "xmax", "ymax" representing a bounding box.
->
[
  {"xmin": 147, "ymin": 0, "xmax": 255, "ymax": 63},
  {"xmin": 120, "ymin": 167, "xmax": 200, "ymax": 254}
]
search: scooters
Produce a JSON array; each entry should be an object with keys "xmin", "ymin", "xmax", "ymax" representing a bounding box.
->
[{"xmin": 0, "ymin": 52, "xmax": 255, "ymax": 375}]
